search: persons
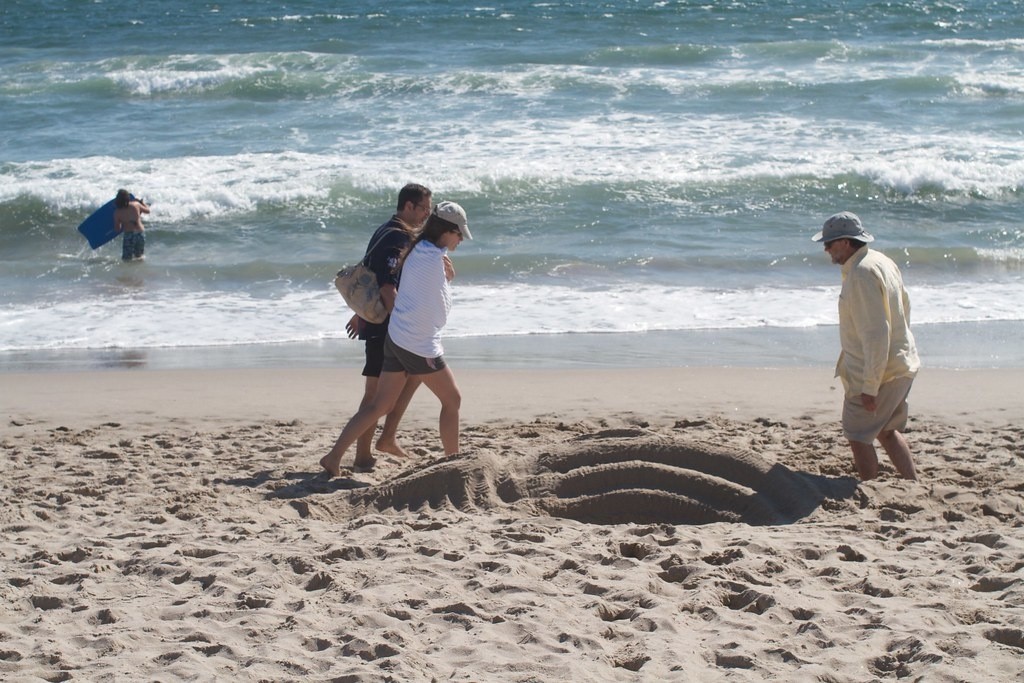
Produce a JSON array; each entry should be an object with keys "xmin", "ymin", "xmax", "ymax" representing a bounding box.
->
[
  {"xmin": 115, "ymin": 189, "xmax": 150, "ymax": 259},
  {"xmin": 345, "ymin": 185, "xmax": 432, "ymax": 469},
  {"xmin": 811, "ymin": 211, "xmax": 920, "ymax": 480},
  {"xmin": 320, "ymin": 202, "xmax": 473, "ymax": 477}
]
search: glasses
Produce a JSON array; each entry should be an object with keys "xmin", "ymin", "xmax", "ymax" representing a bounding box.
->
[
  {"xmin": 451, "ymin": 229, "xmax": 463, "ymax": 238},
  {"xmin": 413, "ymin": 201, "xmax": 432, "ymax": 215}
]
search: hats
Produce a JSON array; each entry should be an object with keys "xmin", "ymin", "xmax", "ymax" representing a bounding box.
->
[
  {"xmin": 811, "ymin": 211, "xmax": 874, "ymax": 243},
  {"xmin": 433, "ymin": 201, "xmax": 473, "ymax": 240}
]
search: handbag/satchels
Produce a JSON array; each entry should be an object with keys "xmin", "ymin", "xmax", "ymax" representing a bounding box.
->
[{"xmin": 335, "ymin": 228, "xmax": 404, "ymax": 324}]
